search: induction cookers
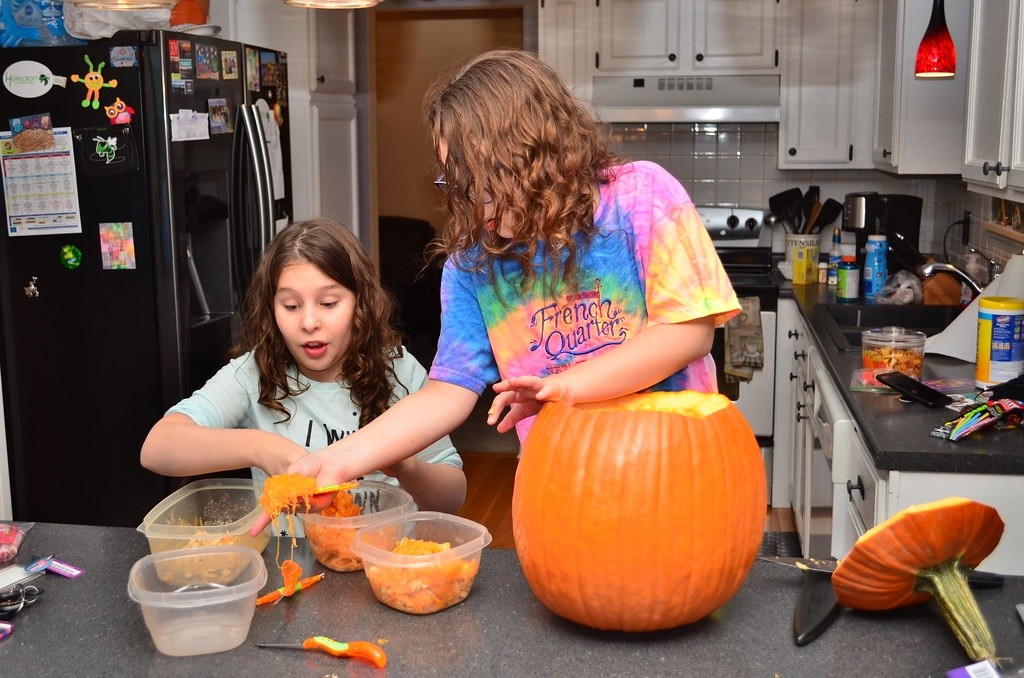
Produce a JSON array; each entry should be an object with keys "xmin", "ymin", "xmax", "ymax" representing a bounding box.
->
[{"xmin": 694, "ymin": 205, "xmax": 779, "ymax": 307}]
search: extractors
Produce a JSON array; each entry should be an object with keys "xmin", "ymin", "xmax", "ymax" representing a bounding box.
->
[{"xmin": 592, "ymin": 76, "xmax": 780, "ymax": 122}]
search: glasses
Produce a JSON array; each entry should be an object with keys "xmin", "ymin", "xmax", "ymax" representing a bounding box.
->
[{"xmin": 434, "ymin": 161, "xmax": 504, "ymax": 204}]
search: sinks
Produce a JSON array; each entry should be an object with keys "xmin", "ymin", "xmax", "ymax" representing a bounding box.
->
[
  {"xmin": 842, "ymin": 329, "xmax": 947, "ymax": 347},
  {"xmin": 825, "ymin": 303, "xmax": 964, "ymax": 329}
]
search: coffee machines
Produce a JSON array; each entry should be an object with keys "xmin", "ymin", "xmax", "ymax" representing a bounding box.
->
[{"xmin": 841, "ymin": 191, "xmax": 923, "ymax": 278}]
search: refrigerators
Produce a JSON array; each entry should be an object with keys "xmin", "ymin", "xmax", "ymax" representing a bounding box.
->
[{"xmin": 0, "ymin": 29, "xmax": 293, "ymax": 528}]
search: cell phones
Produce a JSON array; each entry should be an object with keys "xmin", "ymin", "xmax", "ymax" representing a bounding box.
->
[{"xmin": 876, "ymin": 370, "xmax": 953, "ymax": 409}]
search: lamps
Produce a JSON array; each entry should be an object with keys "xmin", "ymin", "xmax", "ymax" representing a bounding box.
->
[{"xmin": 914, "ymin": 0, "xmax": 958, "ymax": 79}]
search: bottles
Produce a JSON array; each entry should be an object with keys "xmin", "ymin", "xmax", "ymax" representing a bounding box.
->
[
  {"xmin": 864, "ymin": 234, "xmax": 888, "ymax": 298},
  {"xmin": 828, "ymin": 227, "xmax": 844, "ymax": 285},
  {"xmin": 836, "ymin": 256, "xmax": 860, "ymax": 303},
  {"xmin": 818, "ymin": 263, "xmax": 828, "ymax": 282}
]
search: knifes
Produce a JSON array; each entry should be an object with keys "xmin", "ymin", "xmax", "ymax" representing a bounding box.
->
[{"xmin": 760, "ymin": 556, "xmax": 1005, "ymax": 588}]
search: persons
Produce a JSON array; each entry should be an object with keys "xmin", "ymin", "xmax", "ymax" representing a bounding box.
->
[
  {"xmin": 250, "ymin": 49, "xmax": 742, "ymax": 536},
  {"xmin": 263, "ymin": 61, "xmax": 274, "ymax": 83},
  {"xmin": 140, "ymin": 216, "xmax": 468, "ymax": 536},
  {"xmin": 210, "ymin": 105, "xmax": 229, "ymax": 128},
  {"xmin": 225, "ymin": 58, "xmax": 235, "ymax": 73}
]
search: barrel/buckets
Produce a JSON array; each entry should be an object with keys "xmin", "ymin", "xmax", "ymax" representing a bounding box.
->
[{"xmin": 974, "ymin": 296, "xmax": 1024, "ymax": 388}]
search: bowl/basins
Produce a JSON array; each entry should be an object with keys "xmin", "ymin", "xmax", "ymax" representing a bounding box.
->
[
  {"xmin": 349, "ymin": 511, "xmax": 493, "ymax": 614},
  {"xmin": 777, "ymin": 263, "xmax": 792, "ymax": 279},
  {"xmin": 861, "ymin": 326, "xmax": 926, "ymax": 381},
  {"xmin": 137, "ymin": 479, "xmax": 271, "ymax": 584},
  {"xmin": 128, "ymin": 545, "xmax": 268, "ymax": 657},
  {"xmin": 297, "ymin": 480, "xmax": 413, "ymax": 572}
]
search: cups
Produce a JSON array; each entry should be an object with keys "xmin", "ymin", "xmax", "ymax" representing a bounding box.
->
[{"xmin": 785, "ymin": 233, "xmax": 821, "ymax": 261}]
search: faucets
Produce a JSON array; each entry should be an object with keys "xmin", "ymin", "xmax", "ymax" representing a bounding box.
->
[{"xmin": 917, "ymin": 246, "xmax": 1004, "ymax": 295}]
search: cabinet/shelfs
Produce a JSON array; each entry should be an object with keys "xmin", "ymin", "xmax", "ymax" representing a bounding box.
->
[
  {"xmin": 229, "ymin": 0, "xmax": 360, "ymax": 249},
  {"xmin": 768, "ymin": 297, "xmax": 1024, "ymax": 576},
  {"xmin": 591, "ymin": 0, "xmax": 1024, "ymax": 204}
]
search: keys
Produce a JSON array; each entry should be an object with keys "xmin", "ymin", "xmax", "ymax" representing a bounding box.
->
[{"xmin": 0, "ymin": 591, "xmax": 23, "ymax": 621}]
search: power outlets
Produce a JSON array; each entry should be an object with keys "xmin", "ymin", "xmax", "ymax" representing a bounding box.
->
[{"xmin": 962, "ymin": 210, "xmax": 971, "ymax": 245}]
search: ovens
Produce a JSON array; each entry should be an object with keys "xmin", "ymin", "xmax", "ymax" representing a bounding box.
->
[{"xmin": 708, "ymin": 311, "xmax": 774, "ymax": 450}]
